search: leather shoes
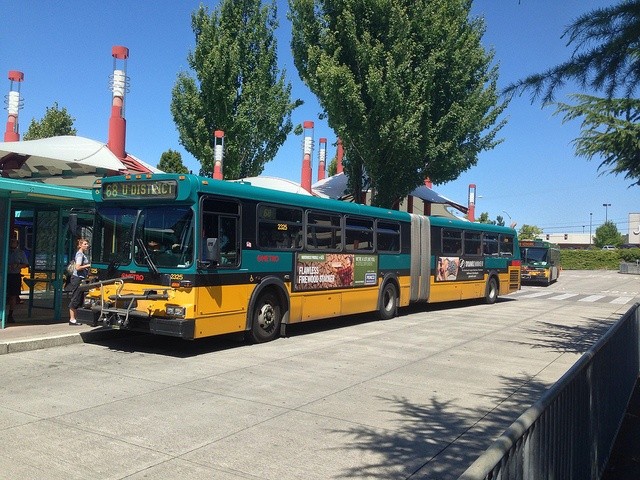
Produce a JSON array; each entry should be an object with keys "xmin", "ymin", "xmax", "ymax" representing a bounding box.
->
[{"xmin": 69, "ymin": 320, "xmax": 82, "ymax": 325}]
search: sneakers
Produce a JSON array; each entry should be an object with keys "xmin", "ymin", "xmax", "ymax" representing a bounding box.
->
[{"xmin": 7, "ymin": 315, "xmax": 15, "ymax": 323}]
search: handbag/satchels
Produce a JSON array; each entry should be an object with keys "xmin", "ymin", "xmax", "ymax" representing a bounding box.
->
[
  {"xmin": 67, "ymin": 259, "xmax": 76, "ymax": 275},
  {"xmin": 77, "ymin": 265, "xmax": 88, "ymax": 276}
]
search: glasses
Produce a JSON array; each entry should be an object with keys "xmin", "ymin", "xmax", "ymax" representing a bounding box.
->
[{"xmin": 10, "ymin": 247, "xmax": 16, "ymax": 249}]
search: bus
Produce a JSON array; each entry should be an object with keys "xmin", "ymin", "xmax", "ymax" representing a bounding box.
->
[
  {"xmin": 73, "ymin": 171, "xmax": 523, "ymax": 354},
  {"xmin": 14, "ymin": 209, "xmax": 97, "ymax": 292},
  {"xmin": 519, "ymin": 238, "xmax": 562, "ymax": 285}
]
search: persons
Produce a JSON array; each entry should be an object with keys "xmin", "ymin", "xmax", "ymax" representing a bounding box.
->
[
  {"xmin": 67, "ymin": 238, "xmax": 90, "ymax": 326},
  {"xmin": 7, "ymin": 236, "xmax": 29, "ymax": 323},
  {"xmin": 213, "ymin": 228, "xmax": 231, "ymax": 253}
]
